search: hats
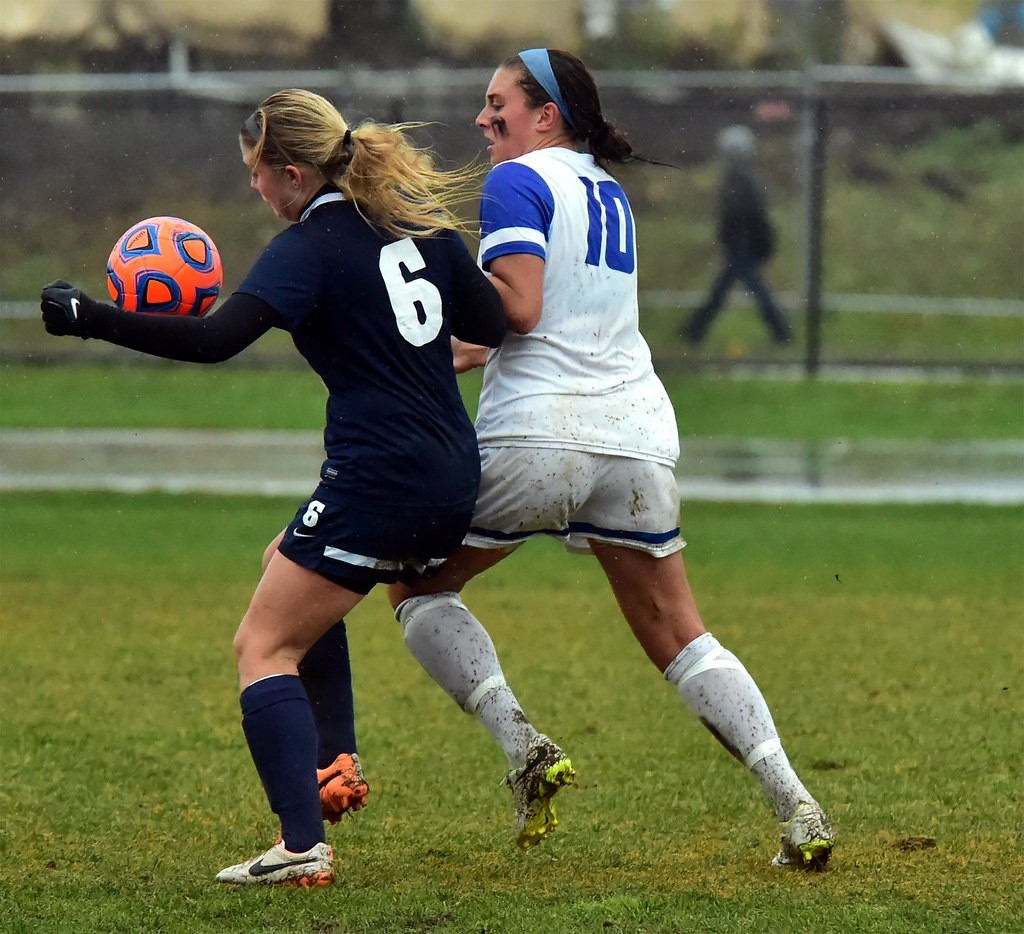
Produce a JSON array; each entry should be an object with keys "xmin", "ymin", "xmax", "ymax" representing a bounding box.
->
[{"xmin": 715, "ymin": 124, "xmax": 758, "ymax": 156}]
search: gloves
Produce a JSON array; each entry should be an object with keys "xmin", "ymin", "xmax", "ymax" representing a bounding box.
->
[{"xmin": 40, "ymin": 278, "xmax": 81, "ymax": 337}]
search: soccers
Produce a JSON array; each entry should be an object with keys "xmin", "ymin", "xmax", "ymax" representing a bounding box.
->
[{"xmin": 104, "ymin": 216, "xmax": 224, "ymax": 318}]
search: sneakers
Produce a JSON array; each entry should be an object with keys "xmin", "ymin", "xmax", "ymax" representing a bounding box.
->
[
  {"xmin": 214, "ymin": 831, "xmax": 336, "ymax": 889},
  {"xmin": 499, "ymin": 734, "xmax": 575, "ymax": 852},
  {"xmin": 274, "ymin": 752, "xmax": 371, "ymax": 845},
  {"xmin": 770, "ymin": 800, "xmax": 835, "ymax": 871}
]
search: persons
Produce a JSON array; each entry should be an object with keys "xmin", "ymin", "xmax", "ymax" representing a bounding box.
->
[
  {"xmin": 670, "ymin": 125, "xmax": 795, "ymax": 349},
  {"xmin": 389, "ymin": 50, "xmax": 835, "ymax": 870},
  {"xmin": 43, "ymin": 91, "xmax": 508, "ymax": 889}
]
